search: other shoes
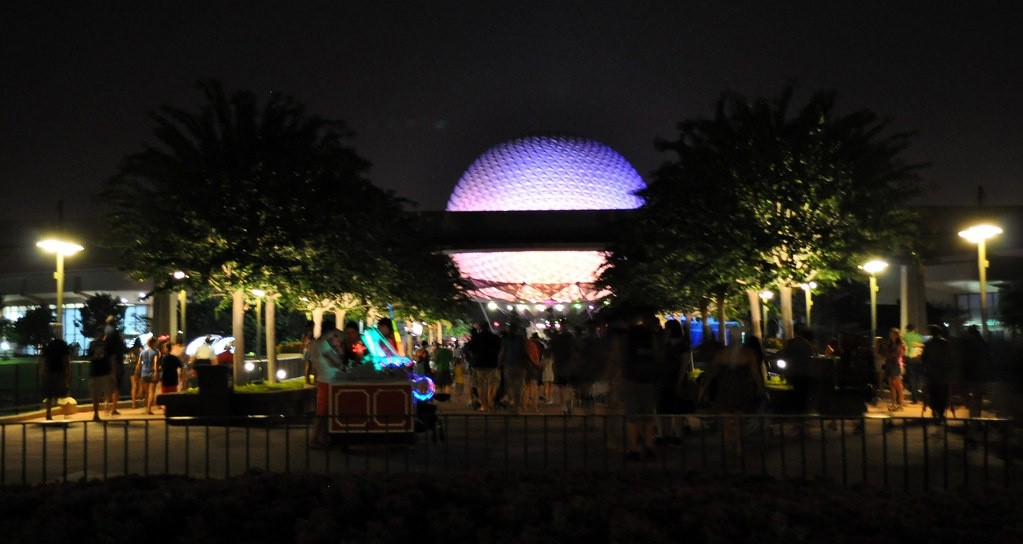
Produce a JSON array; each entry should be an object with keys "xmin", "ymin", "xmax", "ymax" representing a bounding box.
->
[
  {"xmin": 320, "ymin": 442, "xmax": 337, "ymax": 450},
  {"xmin": 671, "ymin": 437, "xmax": 688, "ymax": 448},
  {"xmin": 112, "ymin": 411, "xmax": 119, "ymax": 414},
  {"xmin": 640, "ymin": 451, "xmax": 659, "ymax": 462},
  {"xmin": 736, "ymin": 447, "xmax": 744, "ymax": 460},
  {"xmin": 148, "ymin": 411, "xmax": 154, "ymax": 415},
  {"xmin": 309, "ymin": 441, "xmax": 326, "ymax": 450},
  {"xmin": 620, "ymin": 450, "xmax": 639, "ymax": 461},
  {"xmin": 47, "ymin": 415, "xmax": 52, "ymax": 420},
  {"xmin": 94, "ymin": 415, "xmax": 101, "ymax": 420}
]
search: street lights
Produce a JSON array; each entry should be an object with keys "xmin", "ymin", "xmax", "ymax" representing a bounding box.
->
[
  {"xmin": 759, "ymin": 291, "xmax": 773, "ymax": 347},
  {"xmin": 959, "ymin": 223, "xmax": 1004, "ymax": 345},
  {"xmin": 36, "ymin": 239, "xmax": 84, "ymax": 340},
  {"xmin": 252, "ymin": 289, "xmax": 265, "ymax": 357},
  {"xmin": 175, "ymin": 271, "xmax": 189, "ymax": 346},
  {"xmin": 802, "ymin": 281, "xmax": 817, "ymax": 329},
  {"xmin": 864, "ymin": 259, "xmax": 889, "ymax": 353}
]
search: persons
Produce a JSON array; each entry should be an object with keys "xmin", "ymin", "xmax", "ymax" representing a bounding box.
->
[
  {"xmin": 103, "ymin": 331, "xmax": 133, "ymax": 415},
  {"xmin": 159, "ymin": 344, "xmax": 183, "ymax": 415},
  {"xmin": 377, "ymin": 318, "xmax": 398, "ymax": 356},
  {"xmin": 901, "ymin": 324, "xmax": 925, "ymax": 404},
  {"xmin": 135, "ymin": 336, "xmax": 159, "ymax": 415},
  {"xmin": 87, "ymin": 325, "xmax": 116, "ymax": 421},
  {"xmin": 415, "ymin": 312, "xmax": 877, "ymax": 460},
  {"xmin": 191, "ymin": 337, "xmax": 218, "ymax": 365},
  {"xmin": 301, "ymin": 320, "xmax": 316, "ymax": 385},
  {"xmin": 884, "ymin": 328, "xmax": 907, "ymax": 411},
  {"xmin": 36, "ymin": 327, "xmax": 73, "ymax": 420},
  {"xmin": 128, "ymin": 336, "xmax": 144, "ymax": 408},
  {"xmin": 104, "ymin": 315, "xmax": 119, "ymax": 336},
  {"xmin": 309, "ymin": 319, "xmax": 346, "ymax": 453},
  {"xmin": 216, "ymin": 342, "xmax": 233, "ymax": 365},
  {"xmin": 960, "ymin": 325, "xmax": 988, "ymax": 426},
  {"xmin": 921, "ymin": 325, "xmax": 954, "ymax": 425},
  {"xmin": 340, "ymin": 320, "xmax": 359, "ymax": 356}
]
